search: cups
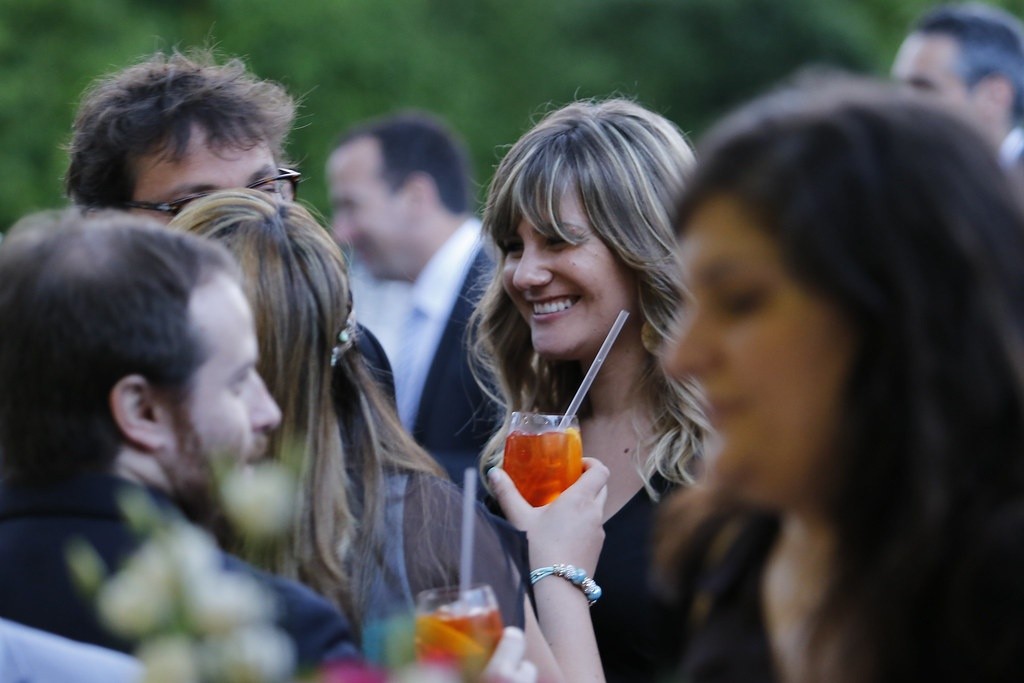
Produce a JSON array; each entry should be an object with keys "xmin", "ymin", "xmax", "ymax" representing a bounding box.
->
[
  {"xmin": 502, "ymin": 409, "xmax": 585, "ymax": 507},
  {"xmin": 414, "ymin": 579, "xmax": 506, "ymax": 682}
]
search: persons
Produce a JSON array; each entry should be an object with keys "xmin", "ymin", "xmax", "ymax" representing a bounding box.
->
[
  {"xmin": 887, "ymin": 15, "xmax": 1024, "ymax": 204},
  {"xmin": 321, "ymin": 122, "xmax": 511, "ymax": 516},
  {"xmin": 63, "ymin": 52, "xmax": 399, "ymax": 501},
  {"xmin": 176, "ymin": 189, "xmax": 611, "ymax": 683},
  {"xmin": 472, "ymin": 99, "xmax": 724, "ymax": 683},
  {"xmin": 621, "ymin": 75, "xmax": 1024, "ymax": 683},
  {"xmin": 0, "ymin": 206, "xmax": 543, "ymax": 683}
]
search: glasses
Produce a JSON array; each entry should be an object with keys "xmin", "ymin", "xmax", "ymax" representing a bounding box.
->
[{"xmin": 126, "ymin": 167, "xmax": 303, "ymax": 218}]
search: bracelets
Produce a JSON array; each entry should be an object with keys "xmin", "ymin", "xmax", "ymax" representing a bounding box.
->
[{"xmin": 530, "ymin": 563, "xmax": 601, "ymax": 607}]
